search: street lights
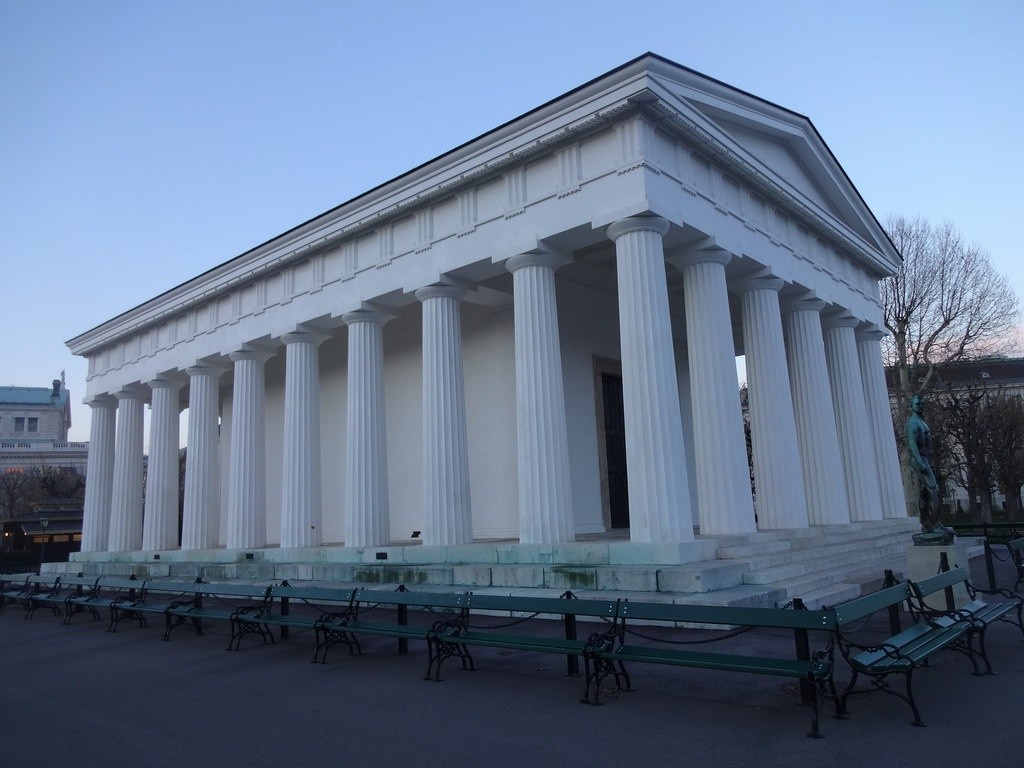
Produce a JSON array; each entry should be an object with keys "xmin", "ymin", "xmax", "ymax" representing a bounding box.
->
[{"xmin": 39, "ymin": 515, "xmax": 49, "ymax": 573}]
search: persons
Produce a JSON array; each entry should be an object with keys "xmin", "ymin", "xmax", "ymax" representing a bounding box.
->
[{"xmin": 907, "ymin": 395, "xmax": 953, "ymax": 533}]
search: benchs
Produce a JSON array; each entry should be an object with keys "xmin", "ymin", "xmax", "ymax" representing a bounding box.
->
[{"xmin": 0, "ymin": 534, "xmax": 1024, "ymax": 740}]
